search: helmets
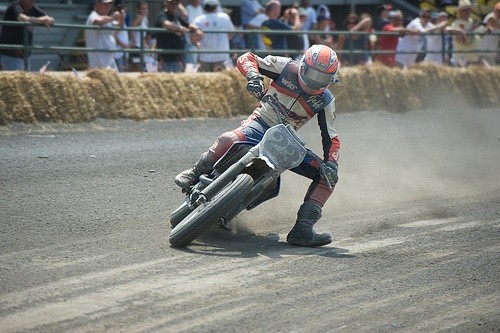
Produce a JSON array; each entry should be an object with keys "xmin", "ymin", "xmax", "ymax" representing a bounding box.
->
[{"xmin": 297, "ymin": 43, "xmax": 342, "ymax": 95}]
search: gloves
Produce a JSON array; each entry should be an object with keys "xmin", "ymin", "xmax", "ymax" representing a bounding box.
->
[
  {"xmin": 246, "ymin": 69, "xmax": 266, "ymax": 99},
  {"xmin": 324, "ymin": 159, "xmax": 339, "ymax": 189}
]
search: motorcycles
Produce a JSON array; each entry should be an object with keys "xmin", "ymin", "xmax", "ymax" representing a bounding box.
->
[{"xmin": 168, "ymin": 86, "xmax": 334, "ymax": 248}]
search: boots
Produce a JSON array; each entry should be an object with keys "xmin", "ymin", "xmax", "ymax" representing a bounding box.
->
[
  {"xmin": 286, "ymin": 201, "xmax": 334, "ymax": 249},
  {"xmin": 172, "ymin": 151, "xmax": 214, "ymax": 190}
]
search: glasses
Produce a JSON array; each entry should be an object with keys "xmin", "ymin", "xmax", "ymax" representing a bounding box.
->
[
  {"xmin": 347, "ymin": 20, "xmax": 357, "ymax": 25},
  {"xmin": 421, "ymin": 14, "xmax": 431, "ymax": 20}
]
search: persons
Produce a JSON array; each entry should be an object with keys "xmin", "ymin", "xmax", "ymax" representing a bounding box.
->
[
  {"xmin": 0, "ymin": 0, "xmax": 500, "ymax": 70},
  {"xmin": 175, "ymin": 44, "xmax": 341, "ymax": 247}
]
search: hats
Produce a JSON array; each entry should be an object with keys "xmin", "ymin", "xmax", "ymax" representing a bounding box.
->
[
  {"xmin": 458, "ymin": 0, "xmax": 473, "ymax": 11},
  {"xmin": 202, "ymin": 0, "xmax": 220, "ymax": 9}
]
